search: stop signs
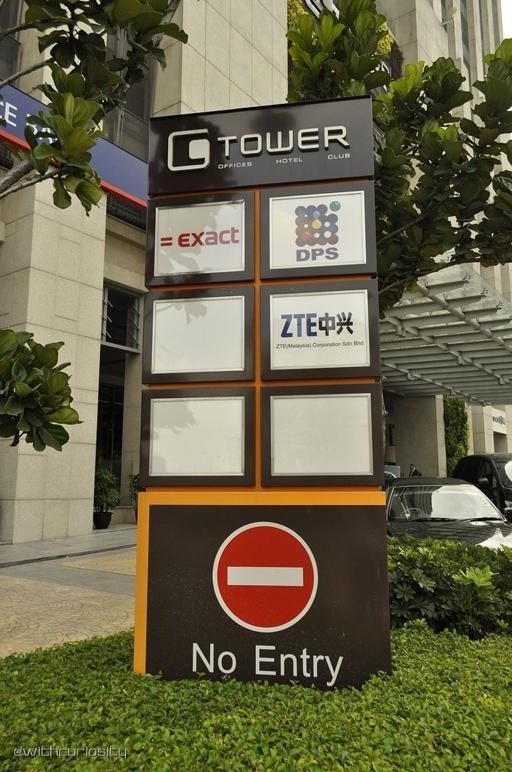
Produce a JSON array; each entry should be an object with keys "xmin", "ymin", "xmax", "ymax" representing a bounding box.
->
[{"xmin": 212, "ymin": 521, "xmax": 320, "ymax": 635}]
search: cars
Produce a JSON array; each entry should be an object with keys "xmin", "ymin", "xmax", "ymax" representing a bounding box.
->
[{"xmin": 385, "ymin": 452, "xmax": 512, "ymax": 553}]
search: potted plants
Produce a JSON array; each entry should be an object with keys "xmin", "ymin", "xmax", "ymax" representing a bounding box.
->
[
  {"xmin": 94, "ymin": 468, "xmax": 120, "ymax": 528},
  {"xmin": 130, "ymin": 472, "xmax": 140, "ymax": 523}
]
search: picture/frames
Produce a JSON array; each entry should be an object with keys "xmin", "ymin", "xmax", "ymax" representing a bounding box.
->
[
  {"xmin": 260, "ymin": 383, "xmax": 385, "ymax": 487},
  {"xmin": 259, "ymin": 281, "xmax": 382, "ymax": 383},
  {"xmin": 258, "ymin": 183, "xmax": 377, "ymax": 279},
  {"xmin": 146, "ymin": 191, "xmax": 254, "ymax": 287},
  {"xmin": 140, "ymin": 388, "xmax": 255, "ymax": 487},
  {"xmin": 142, "ymin": 286, "xmax": 255, "ymax": 384}
]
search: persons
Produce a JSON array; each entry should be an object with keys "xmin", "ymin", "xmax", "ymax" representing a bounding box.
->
[{"xmin": 409, "ymin": 464, "xmax": 422, "ymax": 476}]
